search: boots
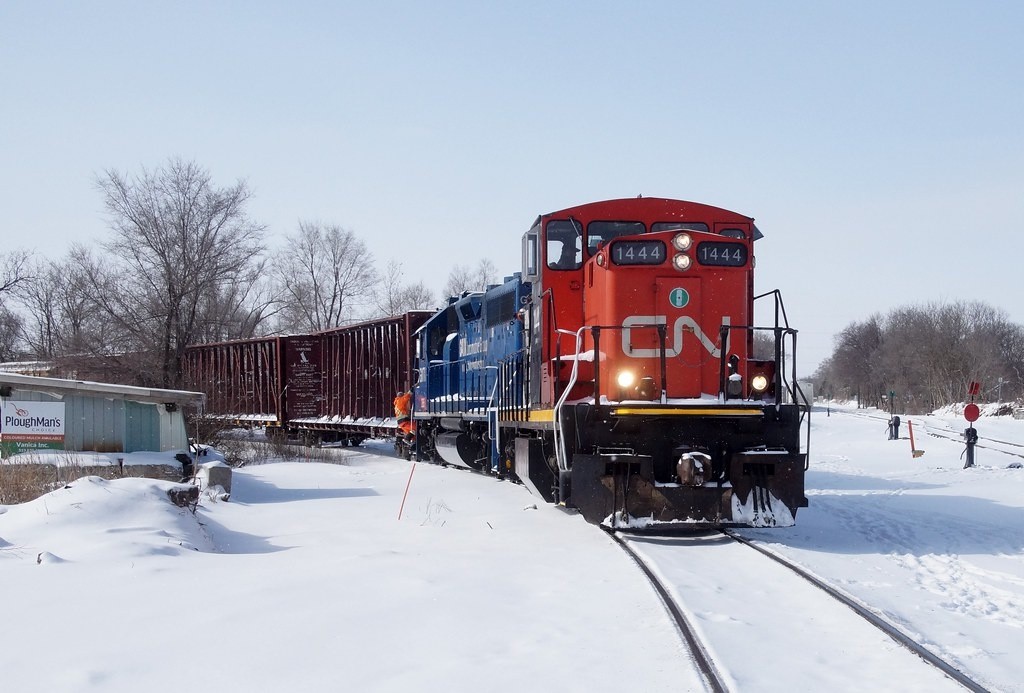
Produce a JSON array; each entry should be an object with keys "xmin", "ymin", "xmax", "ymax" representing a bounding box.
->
[
  {"xmin": 409, "ymin": 440, "xmax": 416, "ymax": 454},
  {"xmin": 402, "ymin": 431, "xmax": 415, "ymax": 445}
]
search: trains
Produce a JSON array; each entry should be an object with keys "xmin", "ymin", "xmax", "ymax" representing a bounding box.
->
[{"xmin": 57, "ymin": 192, "xmax": 814, "ymax": 537}]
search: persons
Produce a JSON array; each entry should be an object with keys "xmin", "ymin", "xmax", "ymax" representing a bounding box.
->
[
  {"xmin": 394, "ymin": 383, "xmax": 419, "ymax": 453},
  {"xmin": 550, "ymin": 244, "xmax": 580, "ymax": 269}
]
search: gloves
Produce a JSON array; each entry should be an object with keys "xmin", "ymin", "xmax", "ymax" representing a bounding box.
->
[{"xmin": 413, "ymin": 383, "xmax": 419, "ymax": 387}]
